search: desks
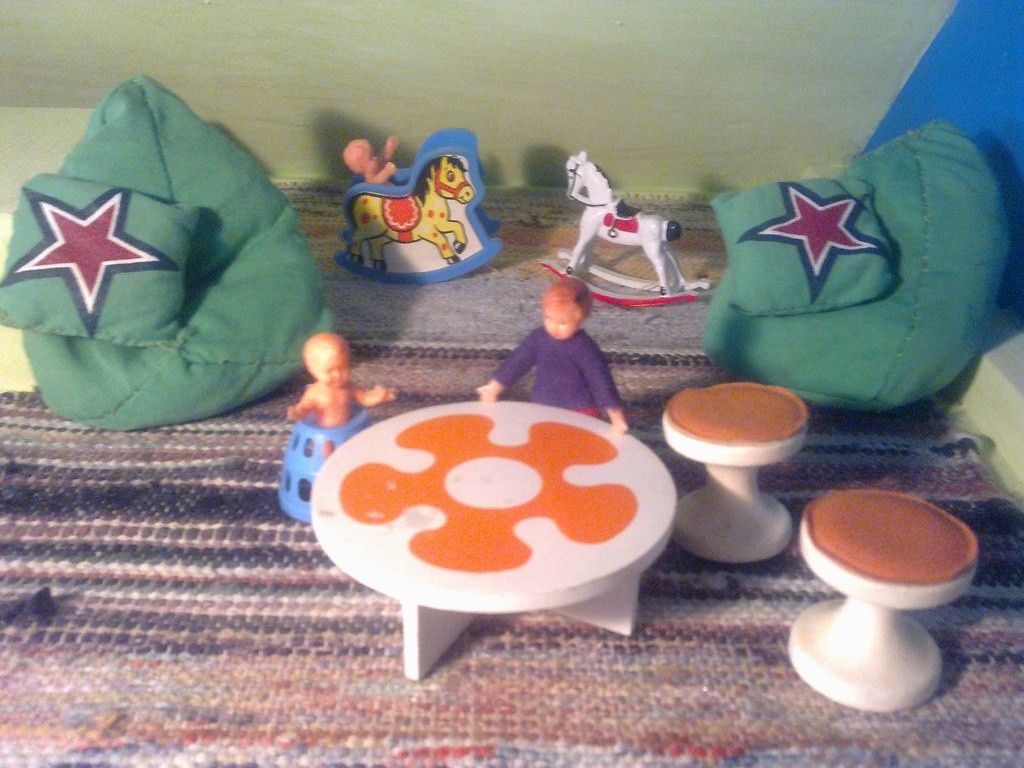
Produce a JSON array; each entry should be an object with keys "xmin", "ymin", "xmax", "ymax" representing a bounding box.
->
[{"xmin": 308, "ymin": 399, "xmax": 679, "ymax": 680}]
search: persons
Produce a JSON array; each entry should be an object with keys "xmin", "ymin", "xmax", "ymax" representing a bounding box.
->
[
  {"xmin": 475, "ymin": 278, "xmax": 630, "ymax": 434},
  {"xmin": 287, "ymin": 333, "xmax": 397, "ymax": 458},
  {"xmin": 343, "ymin": 136, "xmax": 399, "ymax": 186}
]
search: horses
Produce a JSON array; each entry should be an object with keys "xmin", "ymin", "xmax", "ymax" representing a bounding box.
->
[{"xmin": 564, "ymin": 151, "xmax": 686, "ymax": 296}]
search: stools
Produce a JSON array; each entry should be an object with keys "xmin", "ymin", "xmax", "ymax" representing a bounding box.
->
[
  {"xmin": 789, "ymin": 487, "xmax": 979, "ymax": 714},
  {"xmin": 662, "ymin": 383, "xmax": 809, "ymax": 563}
]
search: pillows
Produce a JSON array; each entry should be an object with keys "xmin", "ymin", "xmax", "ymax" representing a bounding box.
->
[
  {"xmin": 711, "ymin": 178, "xmax": 899, "ymax": 318},
  {"xmin": 0, "ymin": 173, "xmax": 201, "ymax": 347}
]
